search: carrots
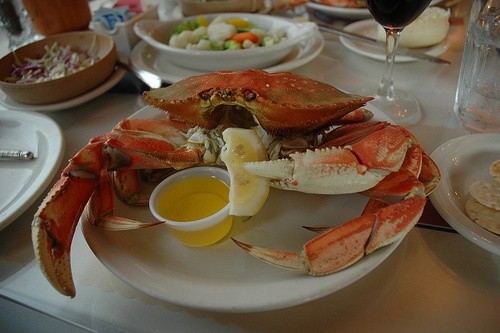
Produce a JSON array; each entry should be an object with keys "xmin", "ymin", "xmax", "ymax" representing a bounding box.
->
[{"xmin": 228, "ymin": 33, "xmax": 259, "ymax": 43}]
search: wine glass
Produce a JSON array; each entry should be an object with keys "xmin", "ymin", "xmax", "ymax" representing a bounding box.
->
[{"xmin": 362, "ymin": 0, "xmax": 433, "ymax": 124}]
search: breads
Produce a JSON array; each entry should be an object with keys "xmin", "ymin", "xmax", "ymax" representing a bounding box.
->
[{"xmin": 379, "ymin": 7, "xmax": 449, "ymax": 46}]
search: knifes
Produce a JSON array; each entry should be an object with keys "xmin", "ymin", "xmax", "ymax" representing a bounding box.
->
[{"xmin": 317, "ymin": 24, "xmax": 450, "ymax": 66}]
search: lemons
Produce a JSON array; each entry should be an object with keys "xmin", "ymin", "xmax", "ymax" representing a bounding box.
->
[{"xmin": 220, "ymin": 129, "xmax": 270, "ymax": 217}]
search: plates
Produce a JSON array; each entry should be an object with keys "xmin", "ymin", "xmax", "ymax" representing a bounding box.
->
[
  {"xmin": 79, "ymin": 100, "xmax": 405, "ymax": 314},
  {"xmin": 339, "ymin": 18, "xmax": 451, "ymax": 63},
  {"xmin": 0, "ymin": 110, "xmax": 67, "ymax": 232},
  {"xmin": 2, "ymin": 52, "xmax": 129, "ymax": 113},
  {"xmin": 304, "ymin": 0, "xmax": 373, "ymax": 21},
  {"xmin": 130, "ymin": 29, "xmax": 325, "ymax": 84},
  {"xmin": 425, "ymin": 132, "xmax": 500, "ymax": 257},
  {"xmin": 132, "ymin": 12, "xmax": 319, "ymax": 74}
]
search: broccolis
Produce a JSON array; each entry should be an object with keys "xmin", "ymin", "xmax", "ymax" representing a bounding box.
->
[{"xmin": 169, "ymin": 15, "xmax": 278, "ymax": 49}]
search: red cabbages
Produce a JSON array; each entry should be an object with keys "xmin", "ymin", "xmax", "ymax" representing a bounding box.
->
[{"xmin": 11, "ymin": 52, "xmax": 84, "ymax": 80}]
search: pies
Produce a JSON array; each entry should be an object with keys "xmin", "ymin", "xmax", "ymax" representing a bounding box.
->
[{"xmin": 465, "ymin": 159, "xmax": 500, "ymax": 237}]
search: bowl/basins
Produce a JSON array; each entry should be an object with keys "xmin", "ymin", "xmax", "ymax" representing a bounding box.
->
[
  {"xmin": 0, "ymin": 30, "xmax": 116, "ymax": 105},
  {"xmin": 148, "ymin": 166, "xmax": 234, "ymax": 247}
]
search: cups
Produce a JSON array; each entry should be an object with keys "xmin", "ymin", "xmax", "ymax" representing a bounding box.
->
[
  {"xmin": 22, "ymin": 0, "xmax": 93, "ymax": 36},
  {"xmin": 452, "ymin": 0, "xmax": 500, "ymax": 132}
]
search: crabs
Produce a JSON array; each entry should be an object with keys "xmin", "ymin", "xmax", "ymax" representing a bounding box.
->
[{"xmin": 31, "ymin": 68, "xmax": 443, "ymax": 299}]
